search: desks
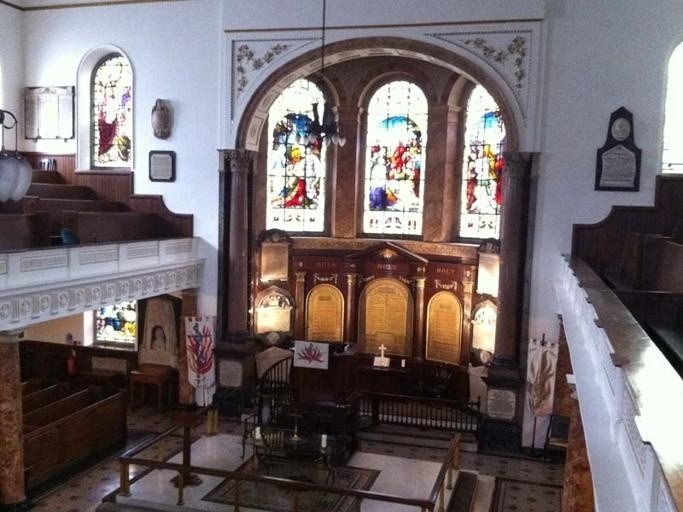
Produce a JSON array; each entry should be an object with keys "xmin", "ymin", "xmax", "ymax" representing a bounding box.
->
[{"xmin": 129, "ymin": 361, "xmax": 175, "ymax": 411}]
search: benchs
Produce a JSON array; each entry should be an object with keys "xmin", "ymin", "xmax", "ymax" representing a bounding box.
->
[
  {"xmin": 21, "ymin": 378, "xmax": 129, "ymax": 492},
  {"xmin": 20, "ymin": 172, "xmax": 133, "ymax": 239}
]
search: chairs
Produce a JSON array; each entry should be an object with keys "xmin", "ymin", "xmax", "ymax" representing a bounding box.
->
[{"xmin": 543, "ymin": 413, "xmax": 570, "ymax": 467}]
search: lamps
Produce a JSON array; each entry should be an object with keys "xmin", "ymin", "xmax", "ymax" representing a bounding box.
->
[{"xmin": 0, "ymin": 109, "xmax": 31, "ymax": 201}]
